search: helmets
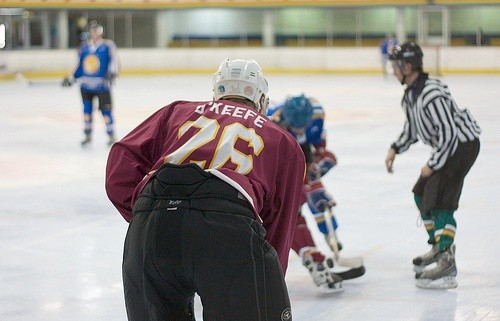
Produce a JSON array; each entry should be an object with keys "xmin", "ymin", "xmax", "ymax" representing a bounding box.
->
[
  {"xmin": 388, "ymin": 41, "xmax": 423, "ymax": 71},
  {"xmin": 212, "ymin": 59, "xmax": 269, "ymax": 116},
  {"xmin": 282, "ymin": 96, "xmax": 312, "ymax": 129}
]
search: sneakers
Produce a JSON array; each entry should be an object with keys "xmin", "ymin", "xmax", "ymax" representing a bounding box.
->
[
  {"xmin": 413, "ymin": 242, "xmax": 439, "ymax": 273},
  {"xmin": 107, "ymin": 133, "xmax": 117, "ymax": 145},
  {"xmin": 414, "ymin": 245, "xmax": 458, "ymax": 290},
  {"xmin": 82, "ymin": 131, "xmax": 91, "ymax": 145},
  {"xmin": 301, "ymin": 249, "xmax": 347, "ymax": 294}
]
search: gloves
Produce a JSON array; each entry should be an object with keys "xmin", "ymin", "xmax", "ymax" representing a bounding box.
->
[{"xmin": 62, "ymin": 76, "xmax": 77, "ymax": 86}]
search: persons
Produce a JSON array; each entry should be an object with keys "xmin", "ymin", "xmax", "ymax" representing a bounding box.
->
[
  {"xmin": 386, "ymin": 41, "xmax": 482, "ymax": 289},
  {"xmin": 382, "ymin": 32, "xmax": 400, "ymax": 77},
  {"xmin": 104, "ymin": 59, "xmax": 307, "ymax": 321},
  {"xmin": 63, "ymin": 24, "xmax": 118, "ymax": 146},
  {"xmin": 266, "ymin": 95, "xmax": 366, "ymax": 298}
]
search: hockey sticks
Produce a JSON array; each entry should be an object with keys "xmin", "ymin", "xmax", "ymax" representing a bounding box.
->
[
  {"xmin": 322, "ymin": 203, "xmax": 363, "ymax": 267},
  {"xmin": 324, "ymin": 267, "xmax": 366, "ymax": 282}
]
